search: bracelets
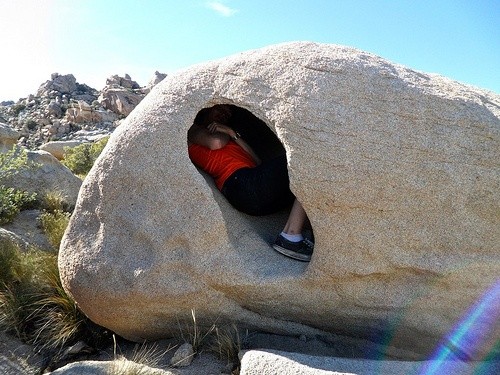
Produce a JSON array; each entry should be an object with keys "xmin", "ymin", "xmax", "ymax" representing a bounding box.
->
[{"xmin": 232, "ymin": 133, "xmax": 240, "ymax": 142}]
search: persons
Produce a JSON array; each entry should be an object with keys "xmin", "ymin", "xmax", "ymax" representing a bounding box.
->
[{"xmin": 186, "ymin": 119, "xmax": 316, "ymax": 262}]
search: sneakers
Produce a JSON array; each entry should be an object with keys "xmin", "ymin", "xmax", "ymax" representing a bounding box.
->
[{"xmin": 272, "ymin": 232, "xmax": 314, "ymax": 263}]
